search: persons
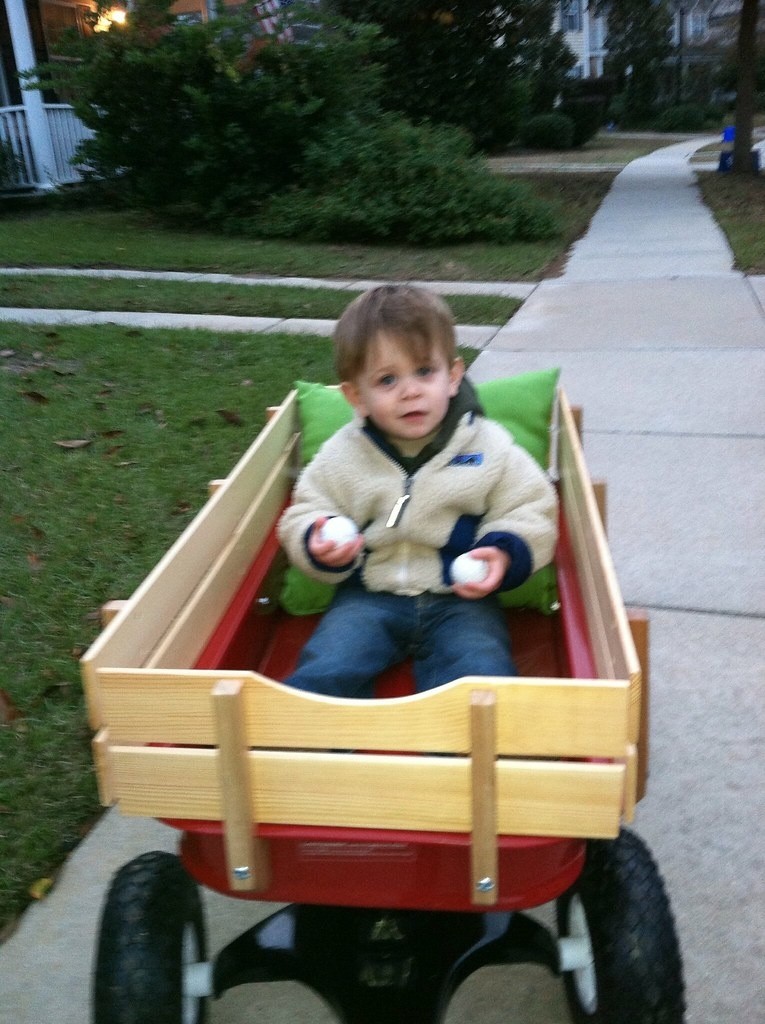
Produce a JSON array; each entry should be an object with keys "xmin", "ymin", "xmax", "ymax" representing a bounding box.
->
[{"xmin": 274, "ymin": 286, "xmax": 562, "ymax": 699}]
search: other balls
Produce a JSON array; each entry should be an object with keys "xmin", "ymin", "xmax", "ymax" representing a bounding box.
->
[
  {"xmin": 450, "ymin": 550, "xmax": 489, "ymax": 585},
  {"xmin": 322, "ymin": 515, "xmax": 358, "ymax": 548}
]
